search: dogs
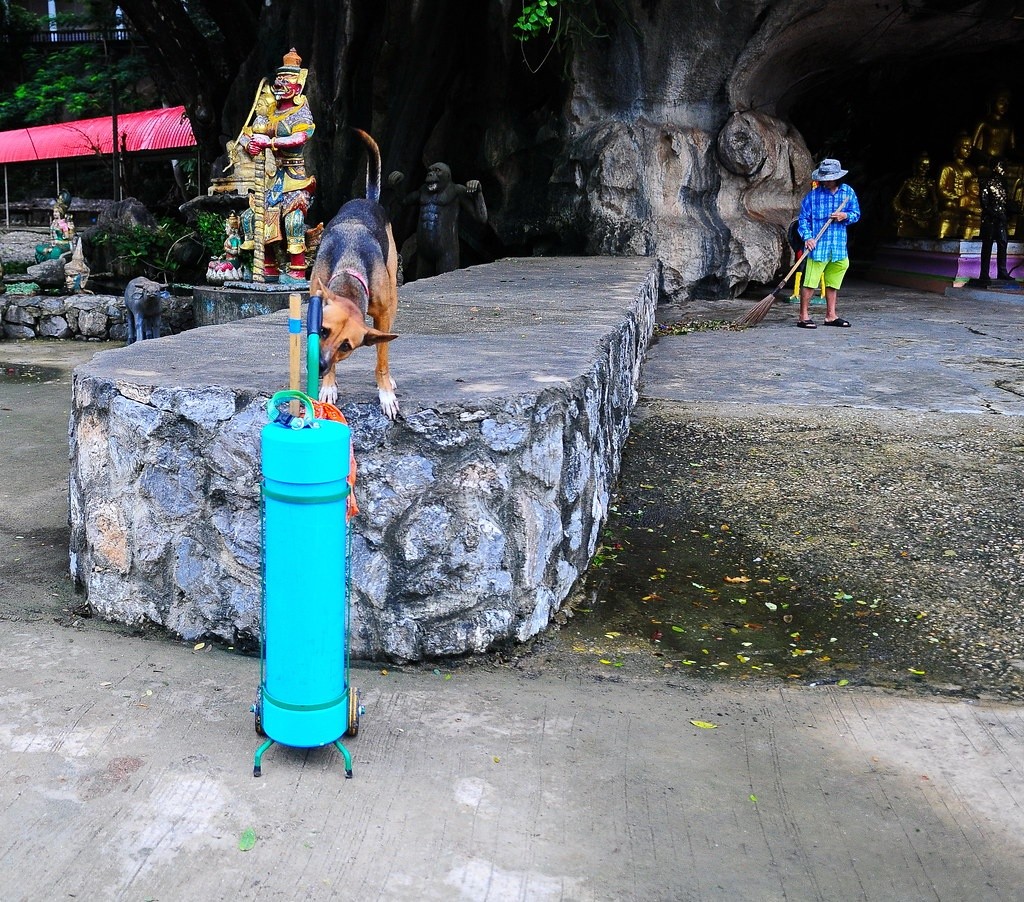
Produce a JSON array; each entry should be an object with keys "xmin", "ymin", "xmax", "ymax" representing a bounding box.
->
[{"xmin": 309, "ymin": 127, "xmax": 399, "ymax": 421}]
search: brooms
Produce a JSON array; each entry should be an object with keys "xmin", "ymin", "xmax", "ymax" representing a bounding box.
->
[{"xmin": 730, "ymin": 197, "xmax": 851, "ymax": 328}]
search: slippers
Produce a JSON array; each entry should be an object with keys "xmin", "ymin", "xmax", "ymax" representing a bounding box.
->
[
  {"xmin": 824, "ymin": 318, "xmax": 852, "ymax": 327},
  {"xmin": 796, "ymin": 319, "xmax": 817, "ymax": 329}
]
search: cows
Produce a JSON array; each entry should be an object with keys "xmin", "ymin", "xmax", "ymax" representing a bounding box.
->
[{"xmin": 125, "ymin": 276, "xmax": 169, "ymax": 344}]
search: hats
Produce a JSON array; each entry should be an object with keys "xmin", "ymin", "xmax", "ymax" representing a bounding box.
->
[{"xmin": 812, "ymin": 158, "xmax": 848, "ymax": 181}]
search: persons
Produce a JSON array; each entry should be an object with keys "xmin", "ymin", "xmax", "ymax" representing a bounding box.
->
[
  {"xmin": 64, "ymin": 237, "xmax": 95, "ymax": 296},
  {"xmin": 796, "ymin": 159, "xmax": 861, "ymax": 329},
  {"xmin": 781, "ymin": 181, "xmax": 827, "ymax": 304},
  {"xmin": 888, "ymin": 85, "xmax": 1024, "ymax": 281},
  {"xmin": 209, "ymin": 213, "xmax": 242, "ymax": 274},
  {"xmin": 34, "ymin": 189, "xmax": 75, "ymax": 265},
  {"xmin": 239, "ymin": 46, "xmax": 317, "ymax": 285}
]
